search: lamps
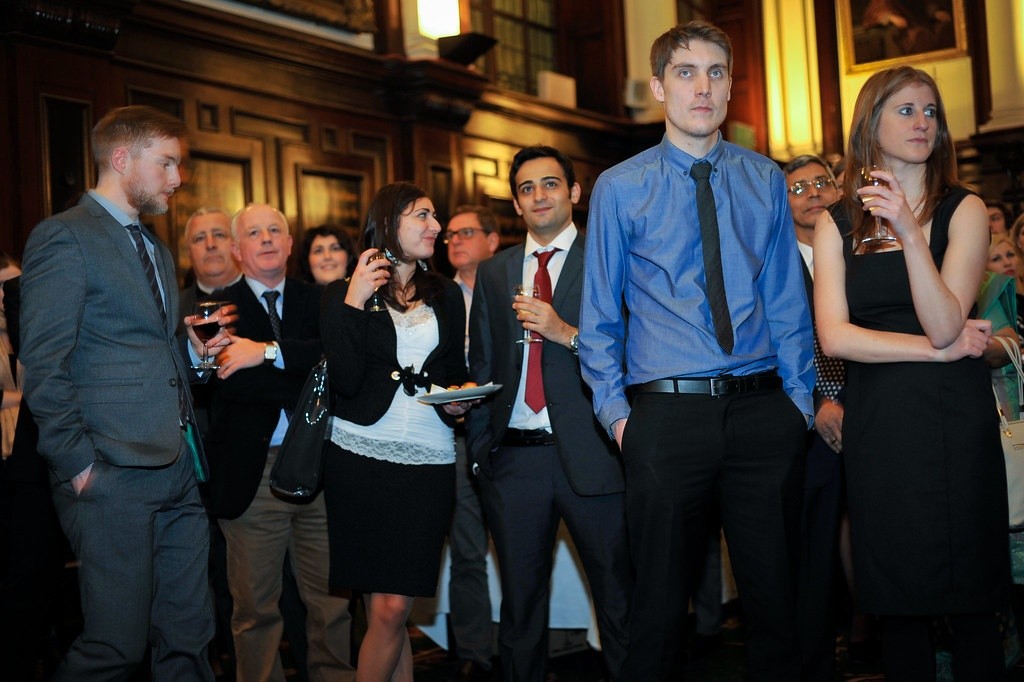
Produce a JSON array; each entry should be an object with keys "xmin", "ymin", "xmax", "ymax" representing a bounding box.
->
[{"xmin": 416, "ymin": 0, "xmax": 461, "ymax": 41}]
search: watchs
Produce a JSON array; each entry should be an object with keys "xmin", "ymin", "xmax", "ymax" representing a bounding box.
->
[
  {"xmin": 571, "ymin": 333, "xmax": 579, "ymax": 354},
  {"xmin": 264, "ymin": 342, "xmax": 277, "ymax": 364}
]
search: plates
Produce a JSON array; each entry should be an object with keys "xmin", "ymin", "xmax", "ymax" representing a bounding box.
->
[{"xmin": 416, "ymin": 385, "xmax": 503, "ymax": 404}]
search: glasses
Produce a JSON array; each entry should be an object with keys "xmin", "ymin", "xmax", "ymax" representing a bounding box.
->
[
  {"xmin": 790, "ymin": 176, "xmax": 834, "ymax": 191},
  {"xmin": 443, "ymin": 228, "xmax": 485, "ymax": 243}
]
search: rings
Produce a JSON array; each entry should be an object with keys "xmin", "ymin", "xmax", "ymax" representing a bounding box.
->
[{"xmin": 833, "ymin": 439, "xmax": 836, "ymax": 444}]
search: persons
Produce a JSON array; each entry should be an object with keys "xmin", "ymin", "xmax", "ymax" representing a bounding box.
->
[
  {"xmin": 774, "ymin": 151, "xmax": 847, "ymax": 682},
  {"xmin": 813, "ymin": 66, "xmax": 1010, "ymax": 682},
  {"xmin": 578, "ymin": 19, "xmax": 813, "ymax": 682},
  {"xmin": 978, "ymin": 203, "xmax": 1024, "ymax": 421},
  {"xmin": 21, "ymin": 106, "xmax": 215, "ymax": 681},
  {"xmin": 320, "ymin": 182, "xmax": 480, "ymax": 681},
  {"xmin": 445, "ymin": 204, "xmax": 497, "ymax": 681},
  {"xmin": 180, "ymin": 204, "xmax": 361, "ymax": 681},
  {"xmin": 454, "ymin": 145, "xmax": 626, "ymax": 681}
]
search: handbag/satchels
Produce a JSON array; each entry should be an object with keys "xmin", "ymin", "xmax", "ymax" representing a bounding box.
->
[
  {"xmin": 269, "ymin": 350, "xmax": 335, "ymax": 504},
  {"xmin": 989, "ymin": 336, "xmax": 1024, "ymax": 527}
]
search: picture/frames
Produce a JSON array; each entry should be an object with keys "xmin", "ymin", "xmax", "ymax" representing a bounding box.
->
[{"xmin": 836, "ymin": 0, "xmax": 969, "ymax": 76}]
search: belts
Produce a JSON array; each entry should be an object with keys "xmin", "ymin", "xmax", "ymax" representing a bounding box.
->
[
  {"xmin": 638, "ymin": 371, "xmax": 777, "ymax": 397},
  {"xmin": 503, "ymin": 429, "xmax": 554, "ymax": 447}
]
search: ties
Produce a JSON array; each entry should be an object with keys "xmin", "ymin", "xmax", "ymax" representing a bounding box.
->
[
  {"xmin": 128, "ymin": 224, "xmax": 167, "ymax": 323},
  {"xmin": 264, "ymin": 290, "xmax": 284, "ymax": 342},
  {"xmin": 524, "ymin": 249, "xmax": 557, "ymax": 414},
  {"xmin": 690, "ymin": 162, "xmax": 734, "ymax": 356},
  {"xmin": 813, "ymin": 319, "xmax": 847, "ymax": 399}
]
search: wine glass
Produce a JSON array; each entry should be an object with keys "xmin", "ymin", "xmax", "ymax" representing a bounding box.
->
[
  {"xmin": 369, "ymin": 252, "xmax": 386, "ymax": 311},
  {"xmin": 860, "ymin": 166, "xmax": 896, "ymax": 244},
  {"xmin": 512, "ymin": 285, "xmax": 543, "ymax": 343},
  {"xmin": 191, "ymin": 302, "xmax": 221, "ymax": 369}
]
